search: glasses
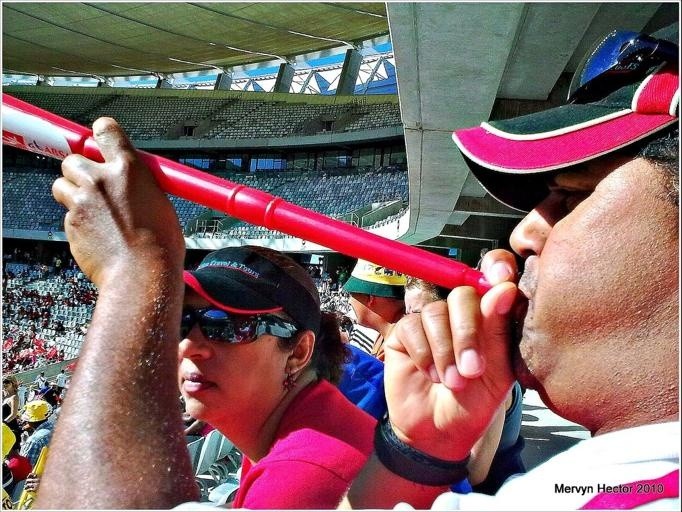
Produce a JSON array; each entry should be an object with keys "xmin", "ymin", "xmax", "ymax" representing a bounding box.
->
[
  {"xmin": 565, "ymin": 19, "xmax": 678, "ymax": 101},
  {"xmin": 177, "ymin": 303, "xmax": 299, "ymax": 344}
]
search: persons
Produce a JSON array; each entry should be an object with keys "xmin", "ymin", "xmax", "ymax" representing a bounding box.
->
[{"xmin": 2, "ymin": 29, "xmax": 679, "ymax": 510}]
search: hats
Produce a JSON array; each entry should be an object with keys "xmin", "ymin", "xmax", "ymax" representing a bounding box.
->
[
  {"xmin": 450, "ymin": 19, "xmax": 678, "ymax": 215},
  {"xmin": 183, "ymin": 246, "xmax": 322, "ymax": 330},
  {"xmin": 21, "ymin": 400, "xmax": 50, "ymax": 422},
  {"xmin": 343, "ymin": 257, "xmax": 410, "ymax": 299}
]
